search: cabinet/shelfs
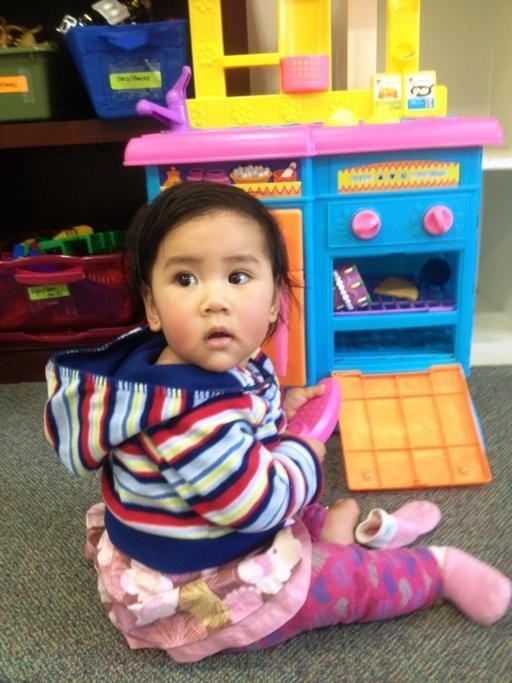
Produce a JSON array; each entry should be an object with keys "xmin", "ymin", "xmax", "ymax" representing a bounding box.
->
[
  {"xmin": 0, "ymin": 111, "xmax": 180, "ymax": 383},
  {"xmin": 120, "ymin": 0, "xmax": 503, "ymax": 401}
]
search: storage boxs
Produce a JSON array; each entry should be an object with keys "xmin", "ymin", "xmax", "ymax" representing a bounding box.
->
[
  {"xmin": 0, "ymin": 243, "xmax": 141, "ymax": 335},
  {"xmin": 0, "ymin": 40, "xmax": 64, "ymax": 124},
  {"xmin": 66, "ymin": 19, "xmax": 190, "ymax": 120}
]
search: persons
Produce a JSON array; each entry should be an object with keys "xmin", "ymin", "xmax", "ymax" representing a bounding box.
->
[{"xmin": 40, "ymin": 178, "xmax": 512, "ymax": 668}]
